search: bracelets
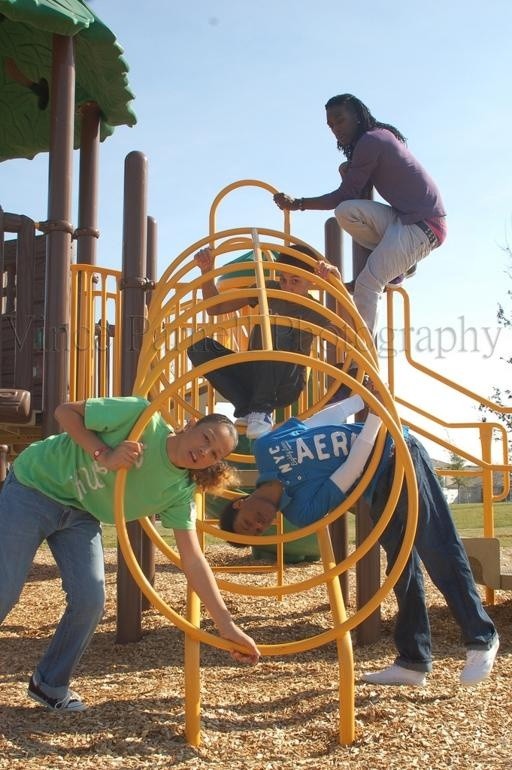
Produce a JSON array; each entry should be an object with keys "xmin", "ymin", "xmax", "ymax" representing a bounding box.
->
[{"xmin": 298, "ymin": 196, "xmax": 305, "ymax": 211}]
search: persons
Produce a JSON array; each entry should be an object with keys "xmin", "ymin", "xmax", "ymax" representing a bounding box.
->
[
  {"xmin": 0, "ymin": 395, "xmax": 262, "ymax": 710},
  {"xmin": 271, "ymin": 92, "xmax": 449, "ymax": 339},
  {"xmin": 218, "ymin": 378, "xmax": 500, "ymax": 687},
  {"xmin": 186, "ymin": 243, "xmax": 344, "ymax": 440}
]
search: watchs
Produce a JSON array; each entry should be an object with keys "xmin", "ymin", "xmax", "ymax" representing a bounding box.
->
[{"xmin": 92, "ymin": 444, "xmax": 109, "ymax": 460}]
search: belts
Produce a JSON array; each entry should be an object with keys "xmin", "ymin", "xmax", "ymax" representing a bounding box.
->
[{"xmin": 416, "ymin": 221, "xmax": 439, "ymax": 250}]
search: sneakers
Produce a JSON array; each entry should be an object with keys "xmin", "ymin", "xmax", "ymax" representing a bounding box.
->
[
  {"xmin": 235, "ymin": 410, "xmax": 273, "ymax": 439},
  {"xmin": 361, "ymin": 666, "xmax": 425, "ymax": 686},
  {"xmin": 387, "ymin": 263, "xmax": 417, "ymax": 288},
  {"xmin": 28, "ymin": 674, "xmax": 87, "ymax": 712},
  {"xmin": 333, "ymin": 369, "xmax": 369, "ymax": 398},
  {"xmin": 460, "ymin": 638, "xmax": 500, "ymax": 685}
]
